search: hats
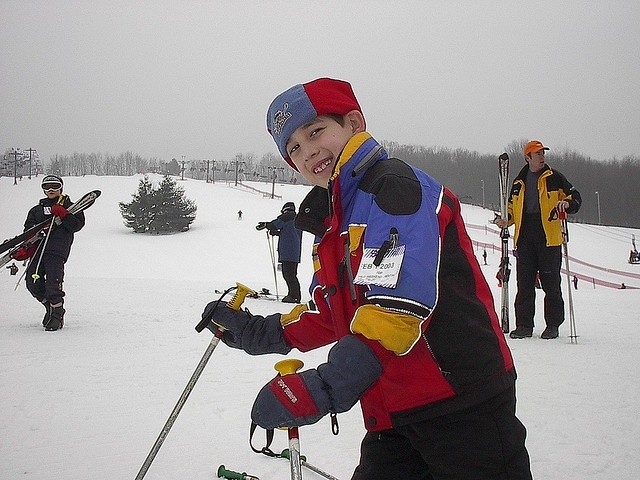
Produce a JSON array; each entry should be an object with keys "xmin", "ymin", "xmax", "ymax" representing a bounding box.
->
[
  {"xmin": 267, "ymin": 78, "xmax": 366, "ymax": 175},
  {"xmin": 42, "ymin": 175, "xmax": 63, "ymax": 194},
  {"xmin": 281, "ymin": 202, "xmax": 295, "ymax": 213},
  {"xmin": 524, "ymin": 141, "xmax": 549, "ymax": 155}
]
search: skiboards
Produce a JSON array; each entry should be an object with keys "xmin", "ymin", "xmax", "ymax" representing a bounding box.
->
[
  {"xmin": 498, "ymin": 153, "xmax": 510, "ymax": 333},
  {"xmin": 0, "ymin": 190, "xmax": 102, "ymax": 268},
  {"xmin": 215, "ymin": 288, "xmax": 284, "ymax": 301}
]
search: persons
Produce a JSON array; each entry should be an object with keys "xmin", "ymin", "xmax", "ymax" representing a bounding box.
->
[
  {"xmin": 572, "ymin": 275, "xmax": 578, "ymax": 290},
  {"xmin": 492, "ymin": 140, "xmax": 582, "ymax": 339},
  {"xmin": 482, "ymin": 250, "xmax": 488, "ymax": 265},
  {"xmin": 255, "ymin": 202, "xmax": 304, "ymax": 302},
  {"xmin": 200, "ymin": 77, "xmax": 534, "ymax": 478},
  {"xmin": 13, "ymin": 176, "xmax": 86, "ymax": 335}
]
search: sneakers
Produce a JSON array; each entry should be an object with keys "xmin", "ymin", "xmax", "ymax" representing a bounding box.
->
[
  {"xmin": 510, "ymin": 325, "xmax": 532, "ymax": 337},
  {"xmin": 283, "ymin": 296, "xmax": 300, "ymax": 304},
  {"xmin": 541, "ymin": 325, "xmax": 558, "ymax": 338},
  {"xmin": 46, "ymin": 301, "xmax": 64, "ymax": 331}
]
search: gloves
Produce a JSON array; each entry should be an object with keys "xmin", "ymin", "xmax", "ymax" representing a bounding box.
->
[
  {"xmin": 251, "ymin": 334, "xmax": 383, "ymax": 430},
  {"xmin": 256, "ymin": 222, "xmax": 267, "ymax": 230},
  {"xmin": 202, "ymin": 300, "xmax": 291, "ymax": 356},
  {"xmin": 50, "ymin": 204, "xmax": 68, "ymax": 220}
]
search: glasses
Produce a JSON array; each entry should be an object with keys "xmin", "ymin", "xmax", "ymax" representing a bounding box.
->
[{"xmin": 41, "ymin": 181, "xmax": 62, "ymax": 191}]
